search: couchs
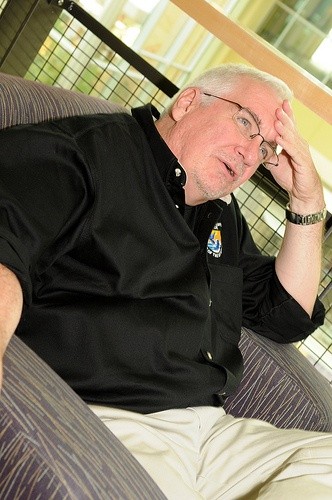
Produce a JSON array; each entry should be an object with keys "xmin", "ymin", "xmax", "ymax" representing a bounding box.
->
[{"xmin": 0, "ymin": 73, "xmax": 332, "ymax": 500}]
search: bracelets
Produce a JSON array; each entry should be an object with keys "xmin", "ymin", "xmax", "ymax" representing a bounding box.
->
[{"xmin": 285, "ymin": 203, "xmax": 328, "ymax": 226}]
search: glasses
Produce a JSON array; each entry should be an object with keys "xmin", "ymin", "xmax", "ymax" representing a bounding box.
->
[{"xmin": 202, "ymin": 92, "xmax": 280, "ymax": 170}]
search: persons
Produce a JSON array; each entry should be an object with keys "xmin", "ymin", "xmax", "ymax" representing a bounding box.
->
[{"xmin": 0, "ymin": 65, "xmax": 331, "ymax": 500}]
